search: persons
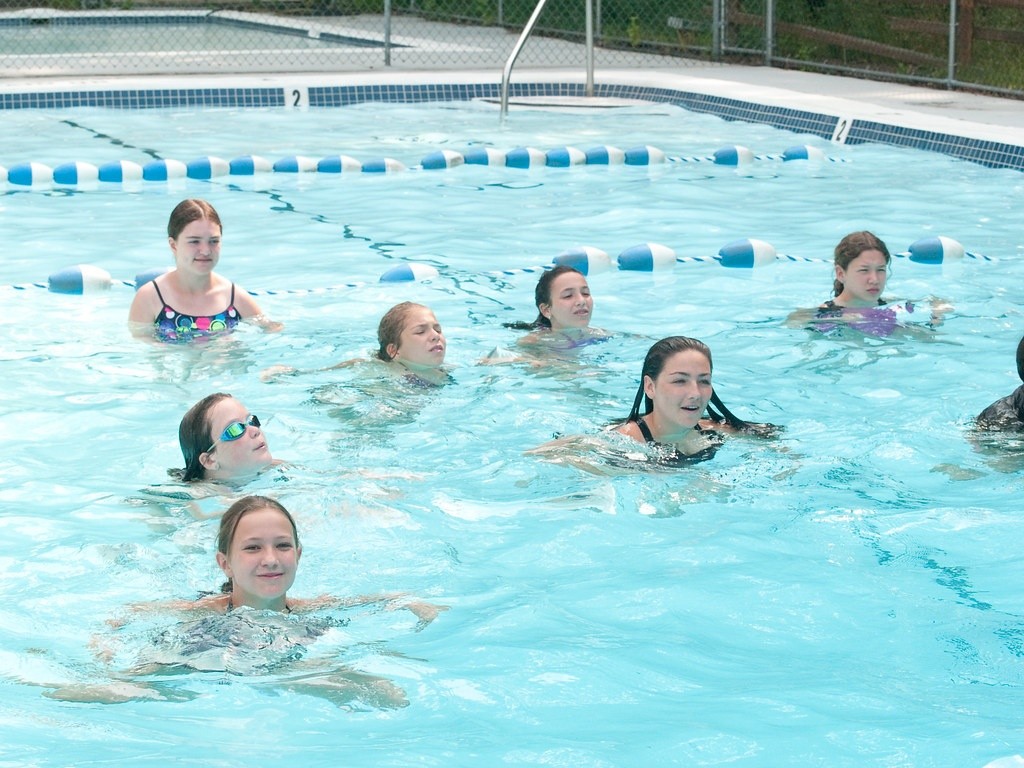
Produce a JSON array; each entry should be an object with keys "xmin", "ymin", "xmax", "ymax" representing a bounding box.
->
[
  {"xmin": 128, "ymin": 198, "xmax": 259, "ymax": 331},
  {"xmin": 977, "ymin": 337, "xmax": 1024, "ymax": 442},
  {"xmin": 375, "ymin": 301, "xmax": 450, "ymax": 376},
  {"xmin": 504, "ymin": 267, "xmax": 608, "ymax": 348},
  {"xmin": 189, "ymin": 497, "xmax": 318, "ymax": 612},
  {"xmin": 177, "ymin": 390, "xmax": 278, "ymax": 481},
  {"xmin": 615, "ymin": 337, "xmax": 783, "ymax": 465},
  {"xmin": 816, "ymin": 229, "xmax": 896, "ymax": 311}
]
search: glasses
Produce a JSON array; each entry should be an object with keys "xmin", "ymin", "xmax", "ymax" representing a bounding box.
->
[{"xmin": 206, "ymin": 414, "xmax": 261, "ymax": 453}]
